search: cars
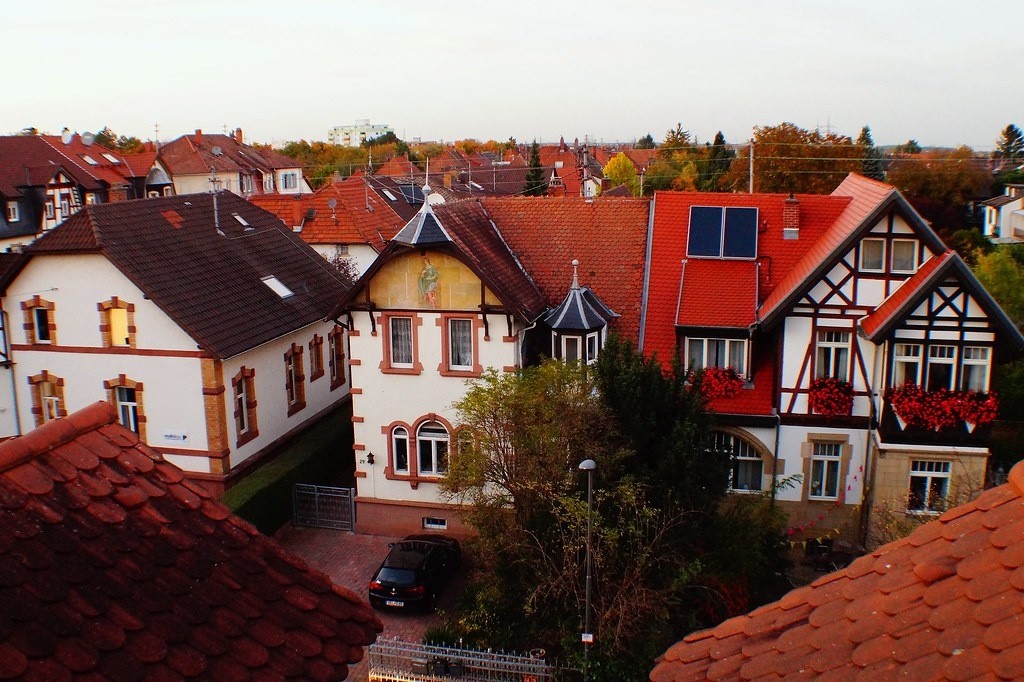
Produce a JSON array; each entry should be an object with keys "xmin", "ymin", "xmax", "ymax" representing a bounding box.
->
[{"xmin": 369, "ymin": 533, "xmax": 462, "ymax": 613}]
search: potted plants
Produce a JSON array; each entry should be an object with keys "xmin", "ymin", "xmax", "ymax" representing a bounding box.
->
[
  {"xmin": 689, "ymin": 359, "xmax": 695, "ymax": 376},
  {"xmin": 738, "ymin": 369, "xmax": 744, "ymax": 379}
]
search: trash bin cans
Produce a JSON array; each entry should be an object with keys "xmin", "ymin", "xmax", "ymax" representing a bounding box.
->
[{"xmin": 529, "ymin": 648, "xmax": 545, "ymax": 672}]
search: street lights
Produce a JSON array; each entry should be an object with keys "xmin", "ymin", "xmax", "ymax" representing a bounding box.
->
[{"xmin": 579, "ymin": 459, "xmax": 597, "ymax": 681}]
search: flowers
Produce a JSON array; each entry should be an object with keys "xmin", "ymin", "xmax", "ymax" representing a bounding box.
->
[
  {"xmin": 922, "ymin": 388, "xmax": 955, "ymax": 432},
  {"xmin": 809, "ymin": 376, "xmax": 856, "ymax": 417},
  {"xmin": 955, "ymin": 388, "xmax": 1000, "ymax": 433},
  {"xmin": 888, "ymin": 380, "xmax": 922, "ymax": 431},
  {"xmin": 701, "ymin": 366, "xmax": 743, "ymax": 403}
]
